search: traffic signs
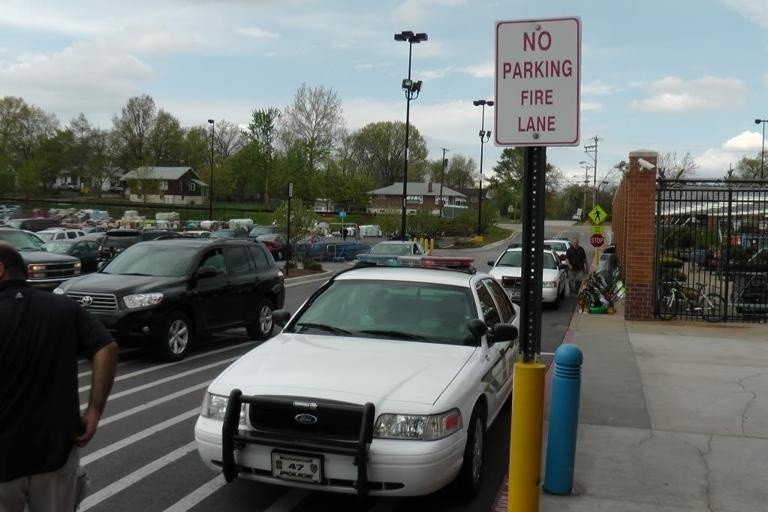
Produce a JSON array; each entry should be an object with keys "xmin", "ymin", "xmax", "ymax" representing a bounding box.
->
[{"xmin": 205, "ymin": 117, "xmax": 216, "ymax": 218}]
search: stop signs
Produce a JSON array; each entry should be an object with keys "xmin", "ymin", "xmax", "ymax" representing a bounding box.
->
[{"xmin": 590, "ymin": 233, "xmax": 605, "ymax": 248}]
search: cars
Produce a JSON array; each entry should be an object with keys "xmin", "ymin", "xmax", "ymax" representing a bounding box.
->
[
  {"xmin": 362, "ymin": 239, "xmax": 430, "ymax": 267},
  {"xmin": 57, "ymin": 181, "xmax": 79, "ymax": 191},
  {"xmin": 107, "ymin": 185, "xmax": 123, "ymax": 193},
  {"xmin": 0, "ymin": 202, "xmax": 286, "ymax": 261},
  {"xmin": 660, "ymin": 245, "xmax": 768, "ymax": 281},
  {"xmin": 486, "ymin": 242, "xmax": 569, "ymax": 310},
  {"xmin": 40, "ymin": 238, "xmax": 103, "ymax": 272},
  {"xmin": 543, "ymin": 236, "xmax": 573, "ymax": 266},
  {"xmin": 572, "ymin": 214, "xmax": 582, "ymax": 221}
]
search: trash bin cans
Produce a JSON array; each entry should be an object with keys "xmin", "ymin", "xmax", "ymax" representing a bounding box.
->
[{"xmin": 599, "ymin": 246, "xmax": 617, "ymax": 272}]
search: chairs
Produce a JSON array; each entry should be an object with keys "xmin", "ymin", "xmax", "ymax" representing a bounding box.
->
[{"xmin": 439, "ymin": 293, "xmax": 468, "ymax": 327}]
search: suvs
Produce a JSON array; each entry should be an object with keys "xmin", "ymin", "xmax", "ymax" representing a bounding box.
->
[
  {"xmin": 52, "ymin": 235, "xmax": 289, "ymax": 359},
  {"xmin": 189, "ymin": 264, "xmax": 522, "ymax": 497},
  {"xmin": 296, "ymin": 222, "xmax": 383, "ymax": 262},
  {"xmin": 93, "ymin": 227, "xmax": 187, "ymax": 274},
  {"xmin": 0, "ymin": 227, "xmax": 83, "ymax": 293}
]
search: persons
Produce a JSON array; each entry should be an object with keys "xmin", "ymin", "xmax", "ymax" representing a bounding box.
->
[
  {"xmin": 565, "ymin": 238, "xmax": 591, "ymax": 298},
  {"xmin": 339, "ymin": 223, "xmax": 349, "ymax": 242},
  {"xmin": 0, "ymin": 241, "xmax": 120, "ymax": 512}
]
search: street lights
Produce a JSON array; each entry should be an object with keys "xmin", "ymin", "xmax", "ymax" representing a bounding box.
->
[
  {"xmin": 578, "ymin": 161, "xmax": 597, "ymax": 211},
  {"xmin": 393, "ymin": 31, "xmax": 430, "ymax": 239},
  {"xmin": 753, "ymin": 118, "xmax": 768, "ymax": 179},
  {"xmin": 597, "ymin": 163, "xmax": 620, "ymax": 194},
  {"xmin": 471, "ymin": 98, "xmax": 496, "ymax": 235},
  {"xmin": 572, "ymin": 174, "xmax": 587, "ymax": 221}
]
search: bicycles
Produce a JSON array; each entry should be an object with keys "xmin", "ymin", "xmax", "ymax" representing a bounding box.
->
[{"xmin": 656, "ymin": 278, "xmax": 725, "ymax": 323}]
